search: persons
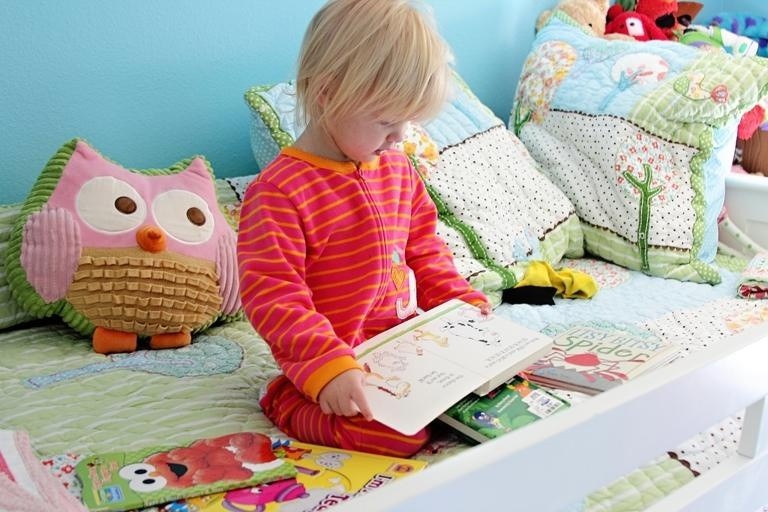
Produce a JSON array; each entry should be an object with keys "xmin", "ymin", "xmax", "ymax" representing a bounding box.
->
[{"xmin": 235, "ymin": 0, "xmax": 494, "ymax": 460}]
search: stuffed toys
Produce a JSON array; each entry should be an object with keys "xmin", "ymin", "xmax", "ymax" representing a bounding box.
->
[{"xmin": 534, "ymin": 1, "xmax": 730, "ymax": 54}]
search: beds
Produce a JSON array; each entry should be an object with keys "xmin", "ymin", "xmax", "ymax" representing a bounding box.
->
[{"xmin": 0, "ymin": 124, "xmax": 768, "ymax": 510}]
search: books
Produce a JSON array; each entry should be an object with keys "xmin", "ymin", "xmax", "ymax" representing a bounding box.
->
[
  {"xmin": 525, "ymin": 313, "xmax": 681, "ymax": 395},
  {"xmin": 474, "ymin": 342, "xmax": 552, "ymax": 397},
  {"xmin": 132, "ymin": 437, "xmax": 430, "ymax": 511},
  {"xmin": 437, "ymin": 376, "xmax": 568, "ymax": 445},
  {"xmin": 328, "ymin": 298, "xmax": 550, "ymax": 435},
  {"xmin": 69, "ymin": 428, "xmax": 295, "ymax": 511}
]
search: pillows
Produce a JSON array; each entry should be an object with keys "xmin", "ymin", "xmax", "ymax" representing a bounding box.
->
[
  {"xmin": 241, "ymin": 56, "xmax": 586, "ymax": 315},
  {"xmin": 8, "ymin": 137, "xmax": 243, "ymax": 356},
  {"xmin": 506, "ymin": 10, "xmax": 768, "ymax": 290}
]
